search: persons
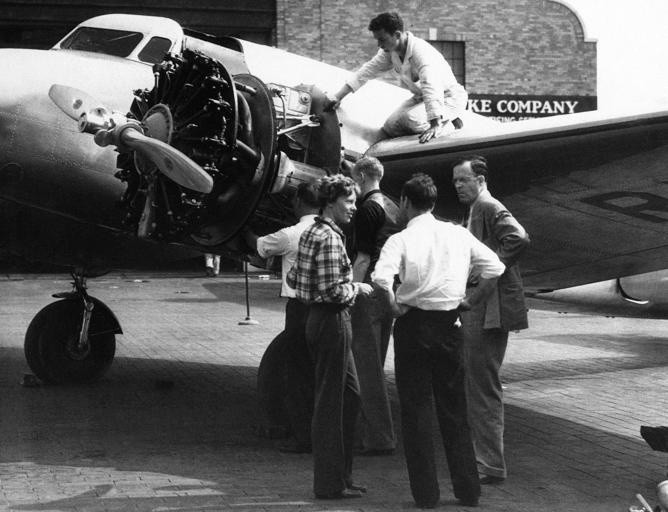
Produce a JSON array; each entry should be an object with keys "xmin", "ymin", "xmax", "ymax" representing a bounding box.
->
[
  {"xmin": 345, "ymin": 156, "xmax": 401, "ymax": 456},
  {"xmin": 287, "ymin": 174, "xmax": 374, "ymax": 500},
  {"xmin": 371, "ymin": 174, "xmax": 506, "ymax": 509},
  {"xmin": 243, "ymin": 178, "xmax": 321, "ymax": 453},
  {"xmin": 452, "ymin": 156, "xmax": 531, "ymax": 486},
  {"xmin": 324, "ymin": 13, "xmax": 469, "ymax": 144},
  {"xmin": 205, "ymin": 254, "xmax": 220, "ymax": 276}
]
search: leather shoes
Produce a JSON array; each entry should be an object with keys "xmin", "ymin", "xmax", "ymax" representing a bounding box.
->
[
  {"xmin": 477, "ymin": 473, "xmax": 501, "ymax": 485},
  {"xmin": 461, "ymin": 499, "xmax": 480, "ymax": 506},
  {"xmin": 362, "ymin": 446, "xmax": 397, "ymax": 457},
  {"xmin": 316, "ymin": 483, "xmax": 368, "ymax": 500},
  {"xmin": 419, "ymin": 501, "xmax": 436, "ymax": 509}
]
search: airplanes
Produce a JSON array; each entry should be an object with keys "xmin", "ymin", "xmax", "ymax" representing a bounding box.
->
[{"xmin": 0, "ymin": 13, "xmax": 668, "ymax": 454}]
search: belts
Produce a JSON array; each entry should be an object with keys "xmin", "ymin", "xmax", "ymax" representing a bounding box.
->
[{"xmin": 466, "ymin": 282, "xmax": 478, "ymax": 287}]
